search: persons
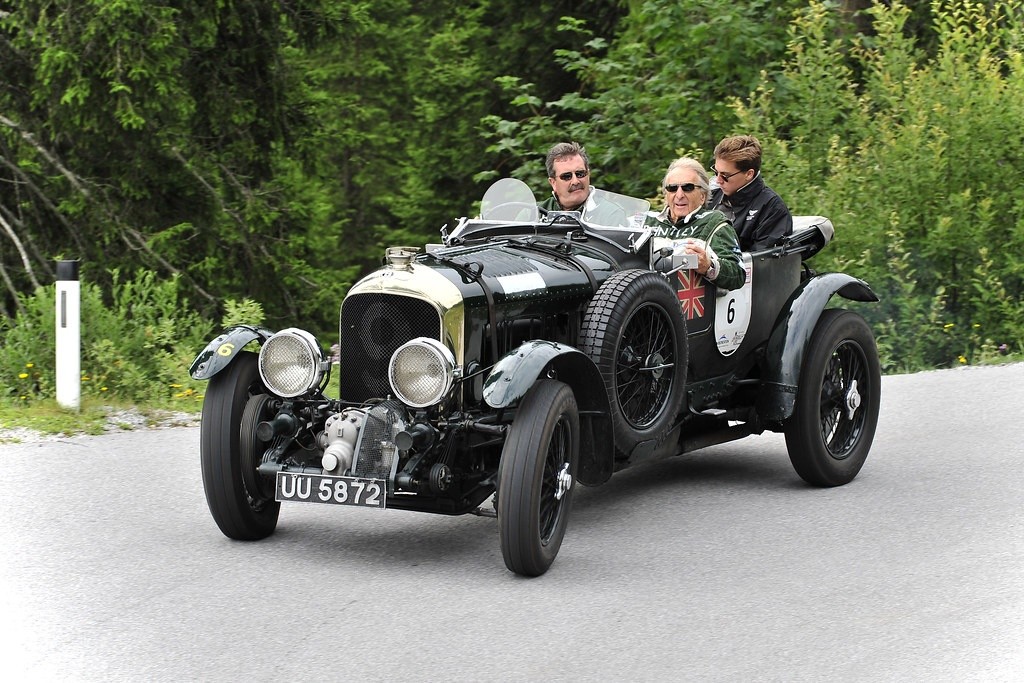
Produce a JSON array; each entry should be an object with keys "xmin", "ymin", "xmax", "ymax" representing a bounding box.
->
[
  {"xmin": 513, "ymin": 142, "xmax": 632, "ymax": 228},
  {"xmin": 702, "ymin": 132, "xmax": 794, "ymax": 255},
  {"xmin": 621, "ymin": 155, "xmax": 746, "ymax": 292}
]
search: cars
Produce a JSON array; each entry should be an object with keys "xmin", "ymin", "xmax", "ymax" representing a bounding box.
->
[{"xmin": 189, "ymin": 176, "xmax": 882, "ymax": 577}]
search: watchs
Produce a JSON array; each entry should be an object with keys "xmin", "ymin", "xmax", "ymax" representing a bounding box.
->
[{"xmin": 705, "ymin": 259, "xmax": 715, "ymax": 280}]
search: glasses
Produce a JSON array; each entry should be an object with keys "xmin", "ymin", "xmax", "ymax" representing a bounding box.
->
[
  {"xmin": 710, "ymin": 164, "xmax": 746, "ymax": 183},
  {"xmin": 663, "ymin": 183, "xmax": 708, "ymax": 192},
  {"xmin": 554, "ymin": 170, "xmax": 588, "ymax": 180}
]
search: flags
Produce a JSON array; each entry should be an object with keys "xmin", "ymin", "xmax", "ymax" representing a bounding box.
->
[{"xmin": 678, "ymin": 269, "xmax": 705, "ymax": 320}]
